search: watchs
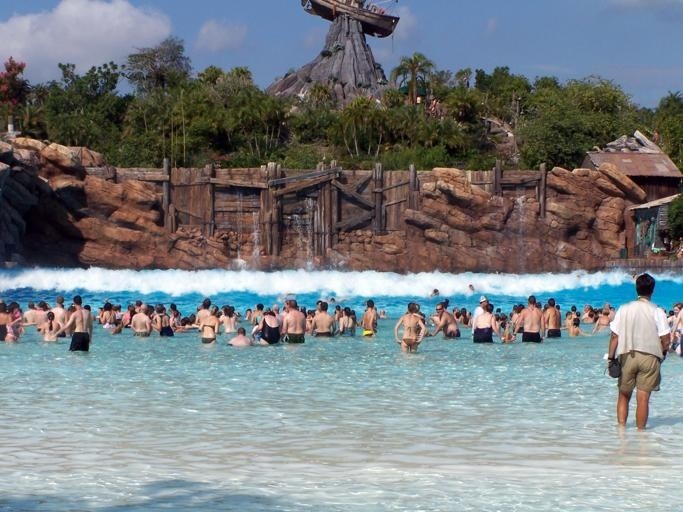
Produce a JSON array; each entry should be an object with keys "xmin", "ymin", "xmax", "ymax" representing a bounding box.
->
[{"xmin": 607, "ymin": 355, "xmax": 614, "ymax": 361}]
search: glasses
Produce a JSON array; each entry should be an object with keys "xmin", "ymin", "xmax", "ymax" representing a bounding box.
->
[{"xmin": 435, "ymin": 308, "xmax": 443, "ymax": 310}]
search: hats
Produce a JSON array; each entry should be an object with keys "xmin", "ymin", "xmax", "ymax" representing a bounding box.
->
[{"xmin": 480, "ymin": 296, "xmax": 487, "ymax": 302}]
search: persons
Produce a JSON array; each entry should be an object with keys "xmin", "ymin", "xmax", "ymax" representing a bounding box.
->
[
  {"xmin": 453, "ymin": 307, "xmax": 474, "ymax": 327},
  {"xmin": 534, "ymin": 301, "xmax": 543, "ymax": 311},
  {"xmin": 395, "ymin": 304, "xmax": 430, "ymax": 353},
  {"xmin": 512, "ymin": 295, "xmax": 546, "ymax": 344},
  {"xmin": 608, "ymin": 274, "xmax": 672, "ymax": 431},
  {"xmin": 474, "ymin": 304, "xmax": 502, "ymax": 344},
  {"xmin": 472, "ymin": 296, "xmax": 489, "ymax": 337},
  {"xmin": 130, "ymin": 305, "xmax": 152, "ymax": 336},
  {"xmin": 227, "ymin": 328, "xmax": 251, "ymax": 345},
  {"xmin": 362, "ymin": 301, "xmax": 378, "ymax": 337},
  {"xmin": 282, "ymin": 301, "xmax": 306, "ymax": 342},
  {"xmin": 195, "ymin": 298, "xmax": 212, "ymax": 327},
  {"xmin": 111, "ymin": 320, "xmax": 125, "ymax": 334},
  {"xmin": 43, "ymin": 312, "xmax": 62, "ymax": 343},
  {"xmin": 495, "ymin": 304, "xmax": 525, "ymax": 344},
  {"xmin": 133, "ymin": 301, "xmax": 144, "ymax": 315},
  {"xmin": 66, "ymin": 303, "xmax": 77, "ymax": 331},
  {"xmin": 265, "ymin": 302, "xmax": 288, "ymax": 321},
  {"xmin": 328, "ymin": 296, "xmax": 346, "ymax": 304},
  {"xmin": 432, "ymin": 304, "xmax": 460, "ymax": 339},
  {"xmin": 37, "ymin": 301, "xmax": 49, "ymax": 330},
  {"xmin": 311, "ymin": 303, "xmax": 336, "ymax": 337},
  {"xmin": 379, "ymin": 310, "xmax": 387, "ymax": 320},
  {"xmin": 251, "ymin": 303, "xmax": 265, "ymax": 326},
  {"xmin": 544, "ymin": 297, "xmax": 561, "ymax": 338},
  {"xmin": 668, "ymin": 302, "xmax": 682, "ymax": 355},
  {"xmin": 564, "ymin": 304, "xmax": 616, "ymax": 336},
  {"xmin": 23, "ymin": 301, "xmax": 39, "ymax": 327},
  {"xmin": 200, "ymin": 305, "xmax": 220, "ymax": 344},
  {"xmin": 99, "ymin": 303, "xmax": 136, "ymax": 327},
  {"xmin": 302, "ymin": 306, "xmax": 315, "ymax": 333},
  {"xmin": 465, "ymin": 284, "xmax": 475, "ymax": 293},
  {"xmin": 53, "ymin": 296, "xmax": 92, "ymax": 352},
  {"xmin": 315, "ymin": 300, "xmax": 323, "ymax": 311},
  {"xmin": 430, "ymin": 289, "xmax": 440, "ymax": 297},
  {"xmin": 218, "ymin": 305, "xmax": 253, "ymax": 332},
  {"xmin": 52, "ymin": 297, "xmax": 68, "ymax": 338},
  {"xmin": 0, "ymin": 302, "xmax": 26, "ymax": 343},
  {"xmin": 83, "ymin": 305, "xmax": 94, "ymax": 323},
  {"xmin": 542, "ymin": 304, "xmax": 551, "ymax": 312},
  {"xmin": 556, "ymin": 303, "xmax": 562, "ymax": 312},
  {"xmin": 256, "ymin": 310, "xmax": 280, "ymax": 344},
  {"xmin": 152, "ymin": 305, "xmax": 199, "ymax": 337},
  {"xmin": 334, "ymin": 305, "xmax": 360, "ymax": 335}
]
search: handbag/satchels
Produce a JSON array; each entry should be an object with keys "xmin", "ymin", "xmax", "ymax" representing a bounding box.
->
[{"xmin": 609, "ymin": 358, "xmax": 622, "ymax": 377}]
